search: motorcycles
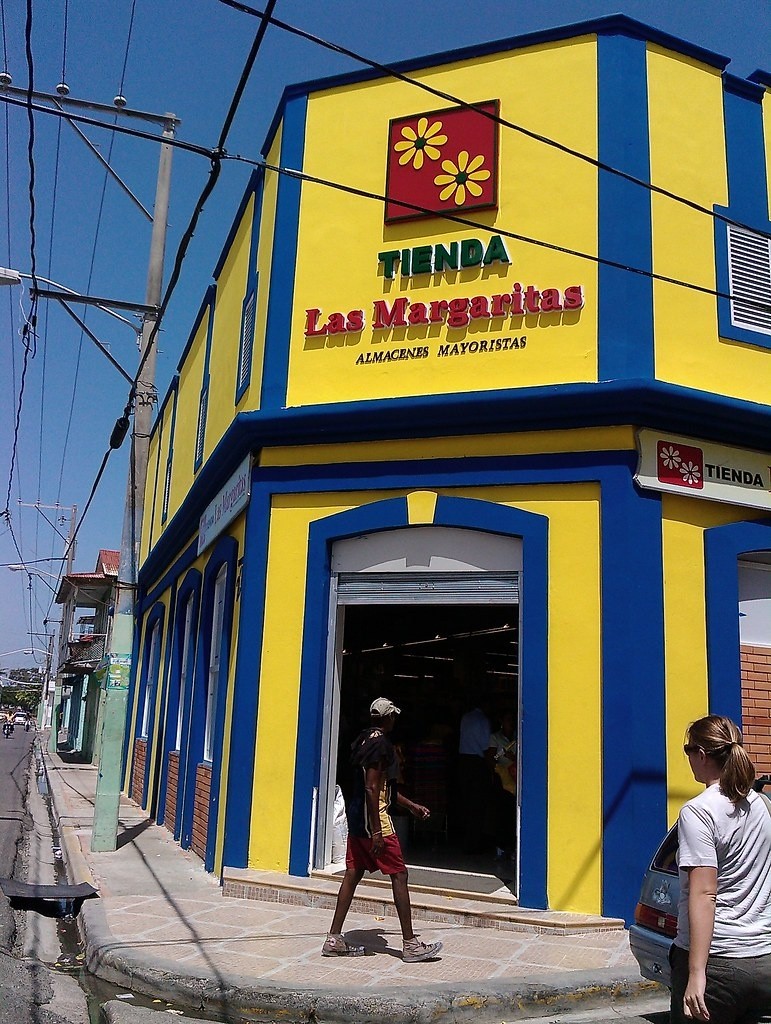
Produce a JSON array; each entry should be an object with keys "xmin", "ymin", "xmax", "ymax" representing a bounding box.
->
[
  {"xmin": 24, "ymin": 717, "xmax": 31, "ymax": 732},
  {"xmin": 4, "ymin": 719, "xmax": 14, "ymax": 739}
]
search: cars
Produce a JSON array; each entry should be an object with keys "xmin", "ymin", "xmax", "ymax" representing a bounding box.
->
[
  {"xmin": 0, "ymin": 711, "xmax": 28, "ymax": 725},
  {"xmin": 628, "ymin": 774, "xmax": 770, "ymax": 989}
]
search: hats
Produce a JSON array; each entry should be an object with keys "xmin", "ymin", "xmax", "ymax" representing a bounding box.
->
[{"xmin": 370, "ymin": 697, "xmax": 401, "ymax": 716}]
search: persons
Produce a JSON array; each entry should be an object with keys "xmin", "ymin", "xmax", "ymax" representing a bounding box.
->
[
  {"xmin": 2, "ymin": 711, "xmax": 17, "ymax": 734},
  {"xmin": 24, "ymin": 713, "xmax": 33, "ymax": 730},
  {"xmin": 669, "ymin": 716, "xmax": 771, "ymax": 1024},
  {"xmin": 459, "ymin": 693, "xmax": 517, "ymax": 855},
  {"xmin": 322, "ymin": 697, "xmax": 444, "ymax": 963}
]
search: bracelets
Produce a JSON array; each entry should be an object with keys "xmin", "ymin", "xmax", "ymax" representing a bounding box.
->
[{"xmin": 373, "ymin": 831, "xmax": 381, "ymax": 835}]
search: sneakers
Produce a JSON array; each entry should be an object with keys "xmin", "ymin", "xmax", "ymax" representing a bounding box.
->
[
  {"xmin": 402, "ymin": 935, "xmax": 442, "ymax": 962},
  {"xmin": 322, "ymin": 933, "xmax": 365, "ymax": 957}
]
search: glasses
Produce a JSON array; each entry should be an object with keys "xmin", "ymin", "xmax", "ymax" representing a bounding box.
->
[{"xmin": 684, "ymin": 745, "xmax": 700, "ymax": 755}]
